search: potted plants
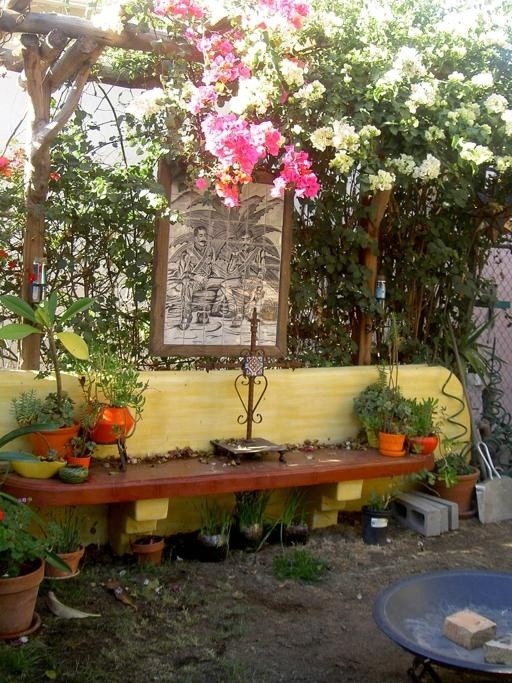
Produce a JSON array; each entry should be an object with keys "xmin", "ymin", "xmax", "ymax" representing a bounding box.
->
[
  {"xmin": 358, "ymin": 496, "xmax": 392, "ymax": 545},
  {"xmin": 431, "ymin": 452, "xmax": 478, "ymax": 516},
  {"xmin": 2, "ymin": 287, "xmax": 144, "ymax": 481},
  {"xmin": 234, "ymin": 491, "xmax": 267, "ymax": 551},
  {"xmin": 349, "ymin": 313, "xmax": 440, "ymax": 457},
  {"xmin": 196, "ymin": 495, "xmax": 230, "ymax": 563},
  {"xmin": 43, "ymin": 506, "xmax": 86, "ymax": 580},
  {"xmin": 278, "ymin": 494, "xmax": 309, "ymax": 544},
  {"xmin": 128, "ymin": 531, "xmax": 164, "ymax": 565},
  {"xmin": 1, "ymin": 498, "xmax": 47, "ymax": 640}
]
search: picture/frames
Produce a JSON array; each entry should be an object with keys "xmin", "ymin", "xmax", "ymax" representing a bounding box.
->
[{"xmin": 149, "ymin": 156, "xmax": 295, "ymax": 358}]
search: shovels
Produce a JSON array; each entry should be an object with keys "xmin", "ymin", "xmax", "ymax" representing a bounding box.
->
[{"xmin": 475, "ymin": 441, "xmax": 512, "ymax": 524}]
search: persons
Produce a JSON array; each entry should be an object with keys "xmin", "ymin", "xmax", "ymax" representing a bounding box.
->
[
  {"xmin": 178, "ymin": 224, "xmax": 228, "ymax": 330},
  {"xmin": 220, "ymin": 229, "xmax": 268, "ymax": 328}
]
